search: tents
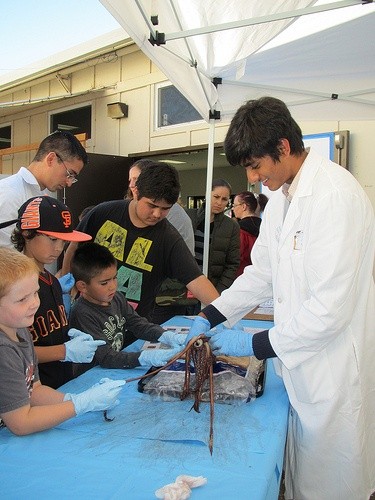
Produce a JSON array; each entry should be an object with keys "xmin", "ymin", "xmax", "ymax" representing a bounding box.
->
[{"xmin": 100, "ymin": 0, "xmax": 375, "ymax": 315}]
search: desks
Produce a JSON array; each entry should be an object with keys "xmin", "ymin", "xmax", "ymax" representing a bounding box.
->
[{"xmin": 0, "ymin": 315, "xmax": 289, "ymax": 500}]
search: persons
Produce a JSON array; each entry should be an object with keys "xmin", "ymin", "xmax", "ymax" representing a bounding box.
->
[
  {"xmin": 256, "ymin": 193, "xmax": 269, "ymax": 220},
  {"xmin": 68, "ymin": 242, "xmax": 189, "ymax": 378},
  {"xmin": 0, "ymin": 247, "xmax": 126, "ymax": 435},
  {"xmin": 124, "ymin": 159, "xmax": 195, "ymax": 326},
  {"xmin": 61, "ymin": 162, "xmax": 245, "ymax": 350},
  {"xmin": 231, "ymin": 192, "xmax": 262, "ymax": 280},
  {"xmin": 79, "ymin": 205, "xmax": 96, "ymax": 221},
  {"xmin": 0, "ymin": 131, "xmax": 88, "ymax": 268},
  {"xmin": 173, "ymin": 179, "xmax": 241, "ymax": 316},
  {"xmin": 10, "ymin": 194, "xmax": 106, "ymax": 390},
  {"xmin": 184, "ymin": 97, "xmax": 375, "ymax": 500}
]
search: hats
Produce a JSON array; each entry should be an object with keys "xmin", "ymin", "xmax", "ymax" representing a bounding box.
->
[{"xmin": 1, "ymin": 195, "xmax": 93, "ymax": 242}]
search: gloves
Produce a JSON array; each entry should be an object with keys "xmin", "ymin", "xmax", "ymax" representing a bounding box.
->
[
  {"xmin": 183, "ymin": 316, "xmax": 211, "ymax": 345},
  {"xmin": 58, "ymin": 273, "xmax": 76, "ymax": 293},
  {"xmin": 61, "ymin": 328, "xmax": 106, "ymax": 364},
  {"xmin": 62, "ymin": 377, "xmax": 127, "ymax": 418},
  {"xmin": 159, "ymin": 330, "xmax": 188, "ymax": 348},
  {"xmin": 137, "ymin": 347, "xmax": 195, "ymax": 369},
  {"xmin": 62, "ymin": 294, "xmax": 77, "ymax": 319},
  {"xmin": 205, "ymin": 330, "xmax": 256, "ymax": 358}
]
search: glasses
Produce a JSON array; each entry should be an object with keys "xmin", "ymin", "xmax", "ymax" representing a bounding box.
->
[
  {"xmin": 232, "ymin": 202, "xmax": 248, "ymax": 208},
  {"xmin": 55, "ymin": 155, "xmax": 78, "ymax": 184}
]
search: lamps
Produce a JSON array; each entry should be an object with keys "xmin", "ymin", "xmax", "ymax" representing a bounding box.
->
[{"xmin": 107, "ymin": 102, "xmax": 128, "ymax": 120}]
